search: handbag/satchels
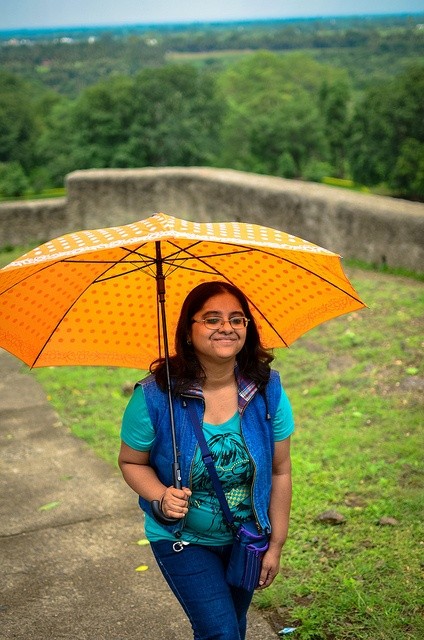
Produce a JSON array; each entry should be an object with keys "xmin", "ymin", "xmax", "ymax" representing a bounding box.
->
[{"xmin": 184, "ymin": 396, "xmax": 271, "ymax": 595}]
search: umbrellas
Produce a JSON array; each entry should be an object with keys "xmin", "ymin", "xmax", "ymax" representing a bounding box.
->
[{"xmin": 0, "ymin": 212, "xmax": 370, "ymax": 526}]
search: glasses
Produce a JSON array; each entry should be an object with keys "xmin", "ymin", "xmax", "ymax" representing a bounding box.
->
[{"xmin": 191, "ymin": 317, "xmax": 251, "ymax": 330}]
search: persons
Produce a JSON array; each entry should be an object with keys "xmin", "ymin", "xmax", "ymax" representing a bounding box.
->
[{"xmin": 116, "ymin": 281, "xmax": 296, "ymax": 636}]
